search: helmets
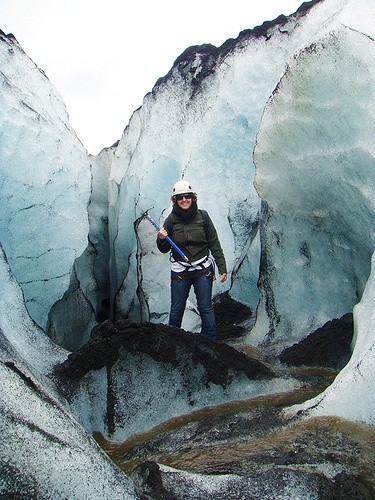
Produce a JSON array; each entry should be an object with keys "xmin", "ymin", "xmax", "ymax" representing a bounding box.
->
[{"xmin": 172, "ymin": 179, "xmax": 195, "ymax": 196}]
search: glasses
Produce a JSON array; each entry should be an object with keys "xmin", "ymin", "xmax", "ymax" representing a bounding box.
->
[{"xmin": 176, "ymin": 194, "xmax": 193, "ymax": 200}]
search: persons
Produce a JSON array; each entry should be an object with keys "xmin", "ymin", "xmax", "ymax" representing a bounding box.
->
[{"xmin": 156, "ymin": 180, "xmax": 228, "ymax": 336}]
